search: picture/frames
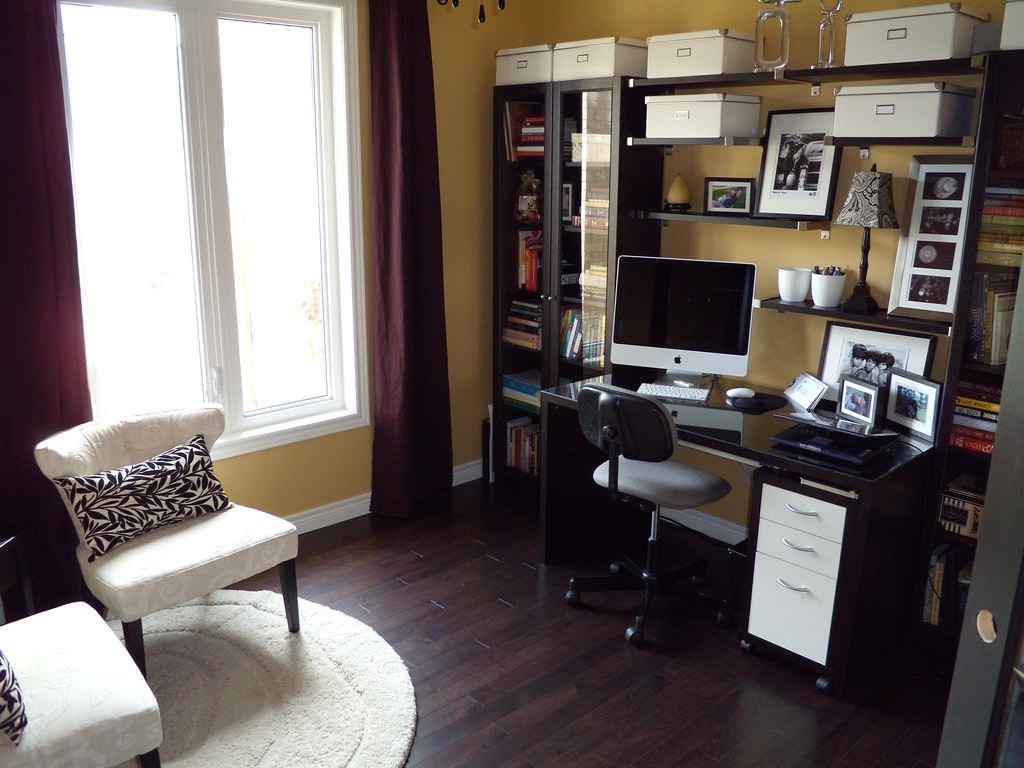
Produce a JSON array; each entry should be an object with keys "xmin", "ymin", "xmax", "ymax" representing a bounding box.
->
[
  {"xmin": 817, "ymin": 320, "xmax": 938, "ymax": 407},
  {"xmin": 703, "ymin": 177, "xmax": 755, "ymax": 217},
  {"xmin": 887, "ymin": 154, "xmax": 975, "ymax": 323},
  {"xmin": 753, "ymin": 107, "xmax": 843, "ymax": 221},
  {"xmin": 560, "ymin": 181, "xmax": 575, "ymax": 224},
  {"xmin": 883, "ymin": 367, "xmax": 943, "ymax": 443},
  {"xmin": 836, "ymin": 374, "xmax": 886, "ymax": 427}
]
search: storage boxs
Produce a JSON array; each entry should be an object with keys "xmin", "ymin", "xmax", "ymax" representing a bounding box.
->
[
  {"xmin": 495, "ymin": 44, "xmax": 553, "ymax": 86},
  {"xmin": 844, "ymin": 2, "xmax": 992, "ymax": 67},
  {"xmin": 834, "ymin": 81, "xmax": 976, "ymax": 136},
  {"xmin": 646, "ymin": 28, "xmax": 755, "ymax": 80},
  {"xmin": 645, "ymin": 92, "xmax": 763, "ymax": 139},
  {"xmin": 553, "ymin": 36, "xmax": 648, "ymax": 82}
]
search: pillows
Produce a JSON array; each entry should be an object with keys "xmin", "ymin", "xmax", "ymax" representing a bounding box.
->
[
  {"xmin": 0, "ymin": 650, "xmax": 25, "ymax": 746},
  {"xmin": 52, "ymin": 433, "xmax": 235, "ymax": 563}
]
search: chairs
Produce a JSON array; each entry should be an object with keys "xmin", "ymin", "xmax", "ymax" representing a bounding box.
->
[
  {"xmin": 565, "ymin": 386, "xmax": 733, "ymax": 643},
  {"xmin": 0, "ymin": 600, "xmax": 163, "ymax": 768},
  {"xmin": 33, "ymin": 403, "xmax": 299, "ymax": 683}
]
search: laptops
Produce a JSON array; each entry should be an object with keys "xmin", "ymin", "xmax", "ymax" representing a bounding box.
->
[{"xmin": 771, "ymin": 412, "xmax": 902, "ymax": 463}]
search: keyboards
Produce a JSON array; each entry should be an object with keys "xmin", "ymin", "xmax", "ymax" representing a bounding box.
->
[{"xmin": 637, "ymin": 383, "xmax": 709, "ymax": 401}]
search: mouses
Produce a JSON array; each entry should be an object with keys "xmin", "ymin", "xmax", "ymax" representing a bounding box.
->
[{"xmin": 726, "ymin": 387, "xmax": 755, "ymax": 398}]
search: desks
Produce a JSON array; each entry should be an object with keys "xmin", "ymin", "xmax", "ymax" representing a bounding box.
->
[
  {"xmin": 540, "ymin": 372, "xmax": 939, "ymax": 713},
  {"xmin": 0, "ymin": 522, "xmax": 36, "ymax": 616}
]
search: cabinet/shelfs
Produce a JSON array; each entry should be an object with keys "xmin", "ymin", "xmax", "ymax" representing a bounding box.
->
[
  {"xmin": 493, "ymin": 75, "xmax": 661, "ymax": 497},
  {"xmin": 539, "ymin": 44, "xmax": 1024, "ymax": 700}
]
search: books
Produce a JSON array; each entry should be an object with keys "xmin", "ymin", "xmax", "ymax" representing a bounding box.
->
[
  {"xmin": 921, "ymin": 545, "xmax": 975, "ymax": 626},
  {"xmin": 502, "ymin": 298, "xmax": 541, "ymax": 350},
  {"xmin": 505, "ymin": 415, "xmax": 540, "ymax": 476},
  {"xmin": 579, "ymin": 263, "xmax": 607, "ymax": 302},
  {"xmin": 967, "ymin": 265, "xmax": 1017, "ymax": 366},
  {"xmin": 949, "ymin": 380, "xmax": 1001, "ymax": 454},
  {"xmin": 572, "ymin": 186, "xmax": 609, "ymax": 229},
  {"xmin": 503, "ymin": 100, "xmax": 575, "ymax": 161},
  {"xmin": 514, "ymin": 228, "xmax": 543, "ymax": 291},
  {"xmin": 559, "ymin": 305, "xmax": 605, "ymax": 368},
  {"xmin": 977, "ymin": 189, "xmax": 1024, "ymax": 269},
  {"xmin": 502, "ymin": 368, "xmax": 540, "ymax": 406},
  {"xmin": 936, "ymin": 472, "xmax": 987, "ymax": 539}
]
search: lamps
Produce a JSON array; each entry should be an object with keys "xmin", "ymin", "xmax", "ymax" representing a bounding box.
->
[{"xmin": 834, "ymin": 162, "xmax": 900, "ymax": 316}]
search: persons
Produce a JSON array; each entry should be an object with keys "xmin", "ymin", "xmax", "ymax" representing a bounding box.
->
[
  {"xmin": 717, "ymin": 190, "xmax": 742, "ymax": 209},
  {"xmin": 838, "ymin": 344, "xmax": 895, "ymax": 387},
  {"xmin": 781, "ymin": 134, "xmax": 807, "ymax": 185},
  {"xmin": 845, "ymin": 394, "xmax": 866, "ymax": 415},
  {"xmin": 897, "ymin": 390, "xmax": 917, "ymax": 421}
]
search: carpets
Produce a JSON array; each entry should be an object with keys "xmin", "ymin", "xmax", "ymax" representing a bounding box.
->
[{"xmin": 105, "ymin": 589, "xmax": 417, "ymax": 768}]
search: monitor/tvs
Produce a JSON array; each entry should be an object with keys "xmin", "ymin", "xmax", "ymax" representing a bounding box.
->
[{"xmin": 610, "ymin": 254, "xmax": 756, "ymax": 388}]
